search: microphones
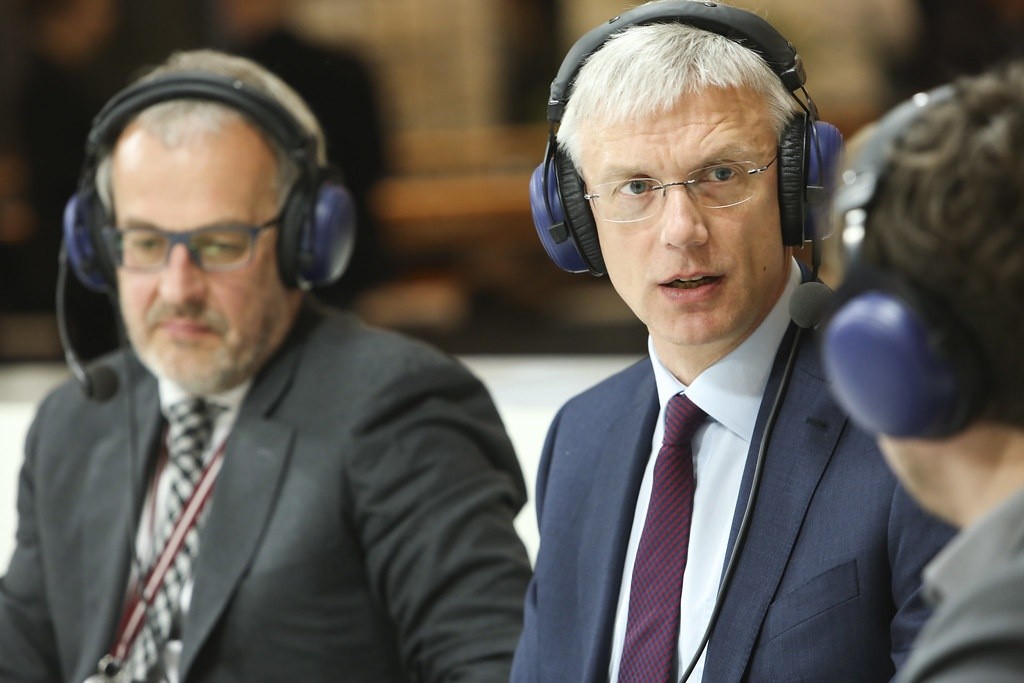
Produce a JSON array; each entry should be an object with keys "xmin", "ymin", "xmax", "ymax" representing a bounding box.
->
[
  {"xmin": 790, "ymin": 264, "xmax": 835, "ymax": 328},
  {"xmin": 56, "ymin": 254, "xmax": 120, "ymax": 401}
]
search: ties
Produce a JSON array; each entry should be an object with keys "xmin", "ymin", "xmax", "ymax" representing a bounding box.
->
[
  {"xmin": 126, "ymin": 397, "xmax": 231, "ymax": 683},
  {"xmin": 618, "ymin": 395, "xmax": 708, "ymax": 683}
]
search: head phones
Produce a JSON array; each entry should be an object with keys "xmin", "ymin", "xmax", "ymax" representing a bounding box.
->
[
  {"xmin": 818, "ymin": 85, "xmax": 988, "ymax": 444},
  {"xmin": 61, "ymin": 71, "xmax": 356, "ymax": 294},
  {"xmin": 527, "ymin": 0, "xmax": 848, "ymax": 278}
]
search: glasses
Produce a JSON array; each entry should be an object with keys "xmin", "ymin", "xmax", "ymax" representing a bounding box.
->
[
  {"xmin": 583, "ymin": 153, "xmax": 777, "ymax": 223},
  {"xmin": 100, "ymin": 218, "xmax": 279, "ymax": 273}
]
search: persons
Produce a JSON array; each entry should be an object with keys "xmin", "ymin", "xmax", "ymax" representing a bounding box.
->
[
  {"xmin": 1, "ymin": 0, "xmax": 401, "ymax": 338},
  {"xmin": 833, "ymin": 62, "xmax": 1024, "ymax": 682},
  {"xmin": 505, "ymin": 0, "xmax": 956, "ymax": 683},
  {"xmin": 0, "ymin": 49, "xmax": 542, "ymax": 683}
]
